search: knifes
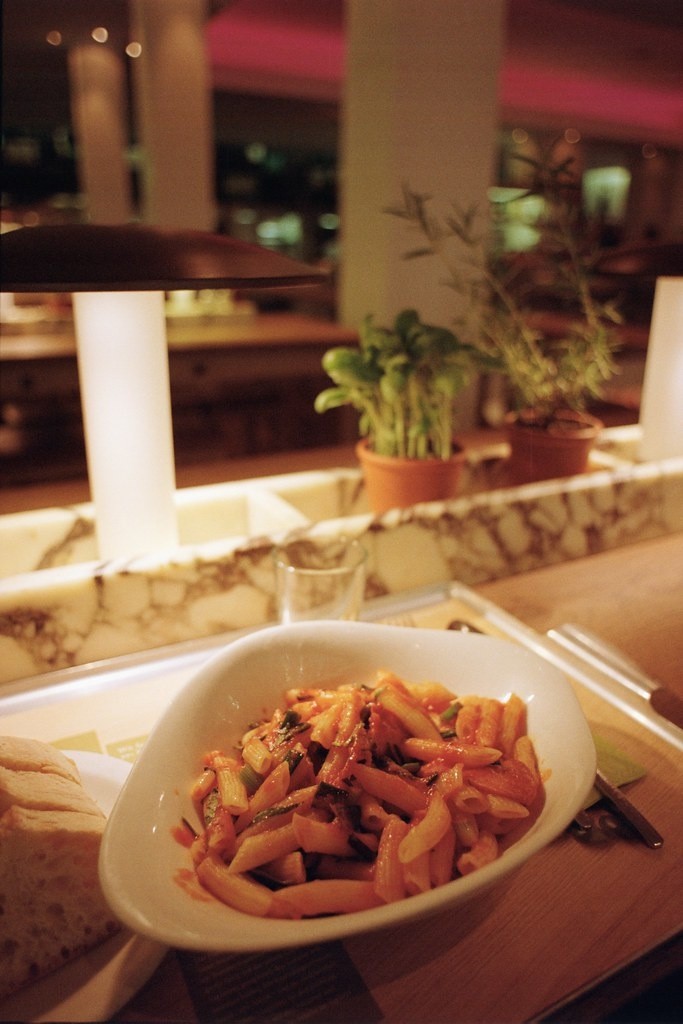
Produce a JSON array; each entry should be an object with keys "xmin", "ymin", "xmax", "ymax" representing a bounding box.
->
[{"xmin": 447, "ymin": 618, "xmax": 664, "ymax": 850}]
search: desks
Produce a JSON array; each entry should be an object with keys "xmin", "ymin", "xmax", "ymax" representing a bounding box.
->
[{"xmin": 0, "ymin": 313, "xmax": 358, "ymax": 454}]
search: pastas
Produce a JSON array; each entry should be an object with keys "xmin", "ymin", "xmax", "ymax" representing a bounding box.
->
[{"xmin": 191, "ymin": 671, "xmax": 542, "ymax": 920}]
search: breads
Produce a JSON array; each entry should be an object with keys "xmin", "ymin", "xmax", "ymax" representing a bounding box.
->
[{"xmin": 0, "ymin": 734, "xmax": 135, "ymax": 1003}]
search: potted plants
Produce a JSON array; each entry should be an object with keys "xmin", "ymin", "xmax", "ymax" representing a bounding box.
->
[
  {"xmin": 312, "ymin": 307, "xmax": 509, "ymax": 515},
  {"xmin": 380, "ymin": 145, "xmax": 625, "ymax": 485}
]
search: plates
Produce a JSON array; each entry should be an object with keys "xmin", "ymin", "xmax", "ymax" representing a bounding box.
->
[{"xmin": 1, "ymin": 748, "xmax": 170, "ymax": 1024}]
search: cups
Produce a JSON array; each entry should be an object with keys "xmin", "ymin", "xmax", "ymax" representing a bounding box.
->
[{"xmin": 276, "ymin": 537, "xmax": 366, "ymax": 624}]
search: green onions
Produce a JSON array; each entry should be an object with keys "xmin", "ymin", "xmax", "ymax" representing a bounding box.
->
[{"xmin": 198, "ymin": 685, "xmax": 466, "ymax": 867}]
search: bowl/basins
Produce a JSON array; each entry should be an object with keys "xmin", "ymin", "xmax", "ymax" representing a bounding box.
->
[{"xmin": 98, "ymin": 619, "xmax": 596, "ymax": 952}]
